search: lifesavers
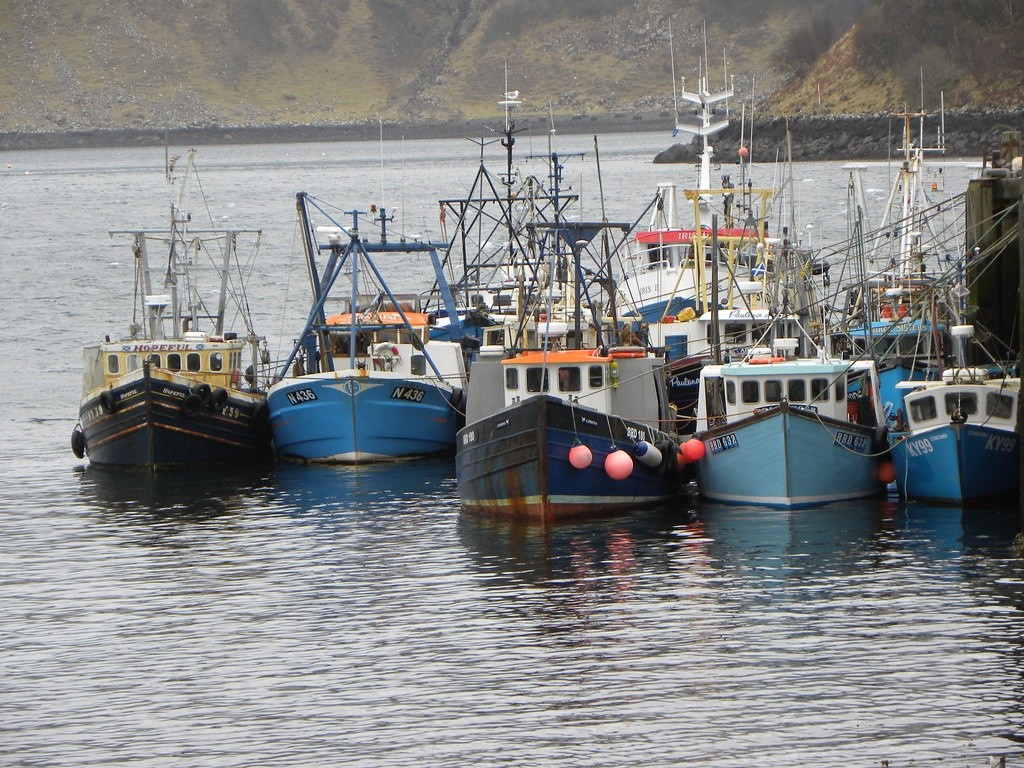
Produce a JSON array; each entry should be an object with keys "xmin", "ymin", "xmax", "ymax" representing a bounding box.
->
[
  {"xmin": 748, "ymin": 356, "xmax": 785, "ymax": 365},
  {"xmin": 100, "ymin": 391, "xmax": 115, "ymax": 414},
  {"xmin": 71, "ymin": 430, "xmax": 84, "ymax": 458},
  {"xmin": 231, "ymin": 369, "xmax": 241, "ymax": 391},
  {"xmin": 608, "ymin": 346, "xmax": 646, "ymax": 359},
  {"xmin": 182, "ymin": 394, "xmax": 204, "ymax": 418},
  {"xmin": 193, "ymin": 383, "xmax": 212, "ymax": 405},
  {"xmin": 208, "ymin": 387, "xmax": 228, "ymax": 412},
  {"xmin": 374, "ymin": 342, "xmax": 400, "ymax": 368}
]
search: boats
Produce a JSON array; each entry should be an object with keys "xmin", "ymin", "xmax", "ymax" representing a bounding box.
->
[
  {"xmin": 77, "ymin": 145, "xmax": 269, "ymax": 476},
  {"xmin": 433, "ymin": 8, "xmax": 974, "ymax": 532},
  {"xmin": 266, "ymin": 189, "xmax": 467, "ymax": 468},
  {"xmin": 890, "ymin": 327, "xmax": 1023, "ymax": 508}
]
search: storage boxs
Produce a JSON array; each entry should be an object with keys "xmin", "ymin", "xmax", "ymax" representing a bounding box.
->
[{"xmin": 662, "ymin": 315, "xmax": 677, "ymax": 323}]
